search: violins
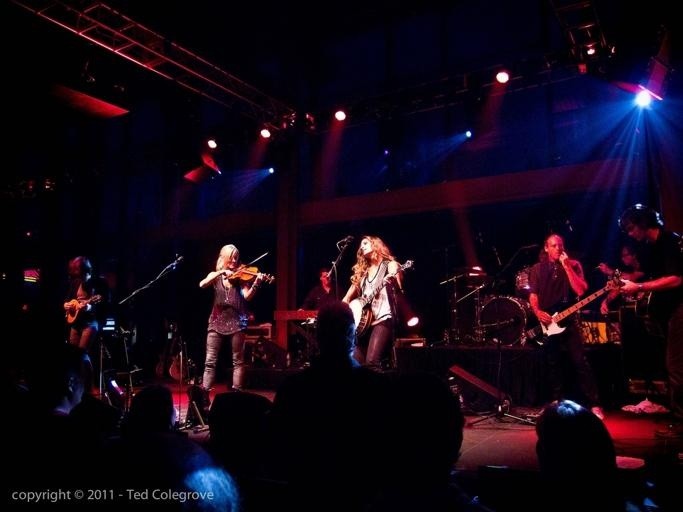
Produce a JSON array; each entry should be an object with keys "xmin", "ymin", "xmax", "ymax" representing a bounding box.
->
[{"xmin": 222, "ymin": 251, "xmax": 275, "ymax": 287}]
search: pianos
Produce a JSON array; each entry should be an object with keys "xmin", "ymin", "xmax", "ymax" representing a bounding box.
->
[{"xmin": 274, "ymin": 311, "xmax": 319, "ymax": 321}]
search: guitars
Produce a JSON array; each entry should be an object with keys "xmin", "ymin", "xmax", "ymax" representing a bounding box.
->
[
  {"xmin": 610, "ymin": 267, "xmax": 657, "ymax": 335},
  {"xmin": 523, "ymin": 281, "xmax": 615, "ymax": 346},
  {"xmin": 64, "ymin": 293, "xmax": 100, "ymax": 323},
  {"xmin": 154, "ymin": 319, "xmax": 172, "ymax": 378},
  {"xmin": 169, "ymin": 323, "xmax": 189, "ymax": 381}
]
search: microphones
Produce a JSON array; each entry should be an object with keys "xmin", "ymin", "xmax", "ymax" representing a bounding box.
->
[
  {"xmin": 491, "ymin": 317, "xmax": 514, "ymax": 326},
  {"xmin": 339, "ymin": 235, "xmax": 353, "ymax": 244},
  {"xmin": 165, "ymin": 256, "xmax": 184, "ymax": 269}
]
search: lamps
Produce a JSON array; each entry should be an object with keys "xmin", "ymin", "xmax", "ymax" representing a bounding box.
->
[
  {"xmin": 639, "ymin": 56, "xmax": 670, "ymax": 102},
  {"xmin": 569, "ymin": 34, "xmax": 615, "ymax": 74}
]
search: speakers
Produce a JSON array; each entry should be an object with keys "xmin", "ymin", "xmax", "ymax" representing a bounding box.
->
[{"xmin": 209, "ymin": 391, "xmax": 273, "ymax": 443}]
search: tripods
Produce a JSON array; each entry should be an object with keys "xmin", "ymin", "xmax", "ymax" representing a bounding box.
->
[{"xmin": 465, "ymin": 328, "xmax": 537, "ymax": 428}]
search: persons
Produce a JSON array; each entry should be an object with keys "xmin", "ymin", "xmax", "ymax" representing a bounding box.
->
[{"xmin": 5, "ymin": 206, "xmax": 682, "ymax": 511}]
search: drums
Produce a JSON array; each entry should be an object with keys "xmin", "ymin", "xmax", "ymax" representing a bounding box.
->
[
  {"xmin": 516, "ymin": 271, "xmax": 531, "ymax": 293},
  {"xmin": 476, "ymin": 294, "xmax": 530, "ymax": 348}
]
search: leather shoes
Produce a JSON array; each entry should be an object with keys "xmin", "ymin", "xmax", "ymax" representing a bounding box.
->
[
  {"xmin": 526, "ymin": 401, "xmax": 559, "ymax": 419},
  {"xmin": 591, "ymin": 406, "xmax": 604, "ymax": 420}
]
